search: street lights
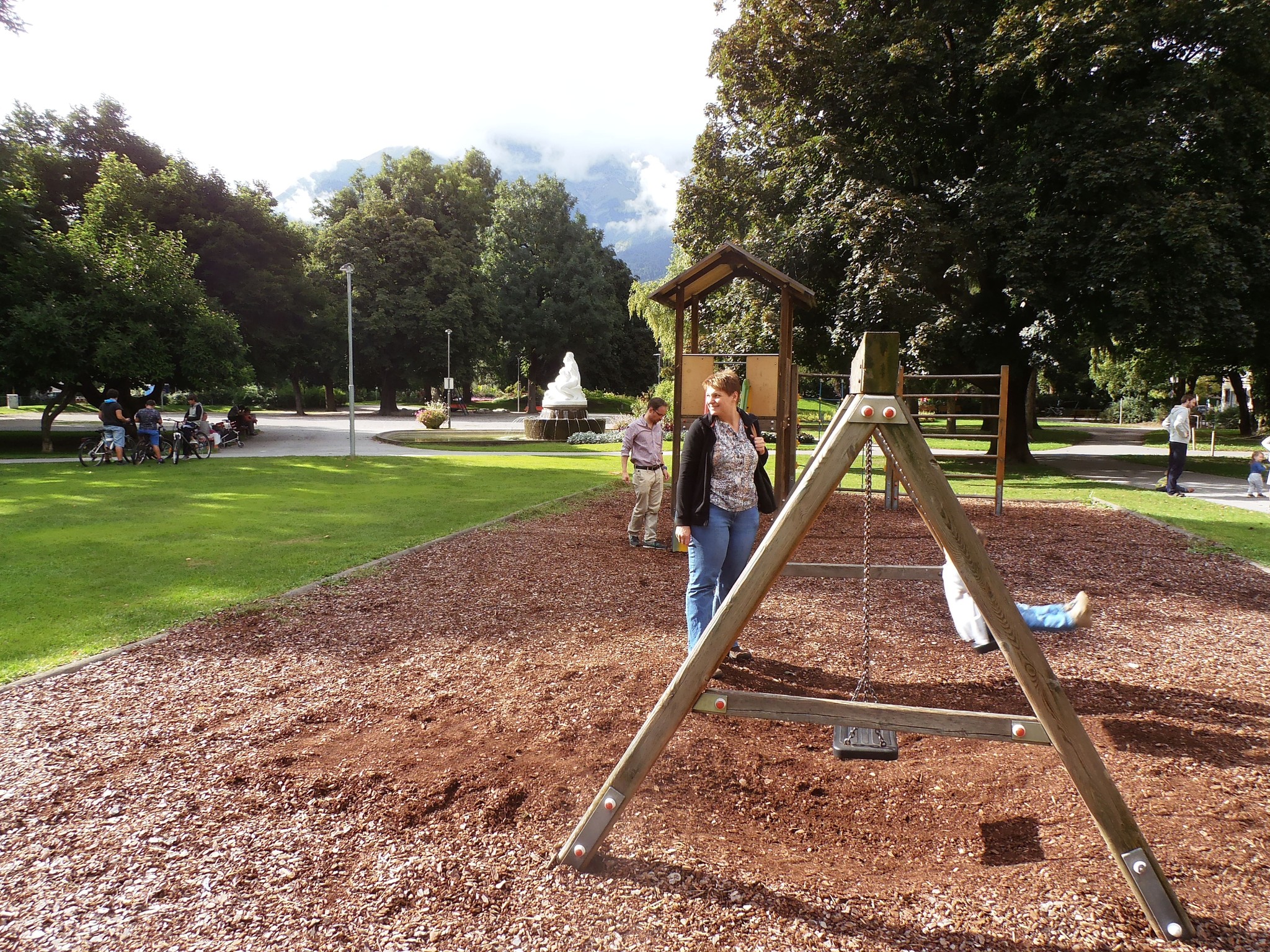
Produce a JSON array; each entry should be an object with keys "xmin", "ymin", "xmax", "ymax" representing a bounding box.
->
[
  {"xmin": 515, "ymin": 355, "xmax": 520, "ymax": 412},
  {"xmin": 339, "ymin": 262, "xmax": 355, "ymax": 457},
  {"xmin": 444, "ymin": 328, "xmax": 453, "ymax": 429}
]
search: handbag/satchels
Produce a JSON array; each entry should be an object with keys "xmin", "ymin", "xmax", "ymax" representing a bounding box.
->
[{"xmin": 753, "ymin": 460, "xmax": 777, "ymax": 514}]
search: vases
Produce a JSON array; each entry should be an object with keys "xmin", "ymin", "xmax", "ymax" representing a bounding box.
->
[{"xmin": 422, "ymin": 418, "xmax": 446, "ymax": 429}]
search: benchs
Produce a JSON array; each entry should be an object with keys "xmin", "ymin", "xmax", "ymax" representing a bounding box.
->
[
  {"xmin": 216, "ymin": 420, "xmax": 248, "ymax": 441},
  {"xmin": 524, "ymin": 406, "xmax": 543, "ymax": 412},
  {"xmin": 445, "ymin": 403, "xmax": 467, "ymax": 412}
]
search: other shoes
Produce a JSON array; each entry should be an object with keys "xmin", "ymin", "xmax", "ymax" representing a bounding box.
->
[
  {"xmin": 1257, "ymin": 494, "xmax": 1266, "ymax": 498},
  {"xmin": 1169, "ymin": 492, "xmax": 1185, "ymax": 497},
  {"xmin": 1247, "ymin": 494, "xmax": 1255, "ymax": 498},
  {"xmin": 1064, "ymin": 590, "xmax": 1093, "ymax": 627},
  {"xmin": 158, "ymin": 458, "xmax": 165, "ymax": 464},
  {"xmin": 117, "ymin": 461, "xmax": 128, "ymax": 466},
  {"xmin": 136, "ymin": 456, "xmax": 147, "ymax": 462},
  {"xmin": 189, "ymin": 439, "xmax": 197, "ymax": 443},
  {"xmin": 182, "ymin": 455, "xmax": 190, "ymax": 461},
  {"xmin": 103, "ymin": 461, "xmax": 114, "ymax": 465}
]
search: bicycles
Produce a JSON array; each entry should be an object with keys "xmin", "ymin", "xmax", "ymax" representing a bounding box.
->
[
  {"xmin": 163, "ymin": 418, "xmax": 211, "ymax": 464},
  {"xmin": 132, "ymin": 424, "xmax": 173, "ymax": 465},
  {"xmin": 77, "ymin": 421, "xmax": 137, "ymax": 467}
]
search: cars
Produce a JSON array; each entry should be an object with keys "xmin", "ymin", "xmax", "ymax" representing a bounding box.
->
[{"xmin": 34, "ymin": 391, "xmax": 79, "ymax": 402}]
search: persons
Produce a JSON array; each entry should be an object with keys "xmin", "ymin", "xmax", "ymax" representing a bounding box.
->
[
  {"xmin": 621, "ymin": 398, "xmax": 670, "ymax": 549},
  {"xmin": 98, "ymin": 389, "xmax": 131, "ymax": 465},
  {"xmin": 242, "ymin": 407, "xmax": 258, "ymax": 436},
  {"xmin": 1162, "ymin": 394, "xmax": 1196, "ymax": 497},
  {"xmin": 1156, "ymin": 470, "xmax": 1195, "ymax": 493},
  {"xmin": 942, "ymin": 523, "xmax": 1091, "ymax": 645},
  {"xmin": 556, "ymin": 352, "xmax": 580, "ymax": 400},
  {"xmin": 198, "ymin": 412, "xmax": 221, "ymax": 450},
  {"xmin": 227, "ymin": 405, "xmax": 245, "ymax": 431},
  {"xmin": 675, "ymin": 369, "xmax": 768, "ymax": 680},
  {"xmin": 1247, "ymin": 451, "xmax": 1268, "ymax": 498},
  {"xmin": 1261, "ymin": 436, "xmax": 1270, "ymax": 486},
  {"xmin": 1257, "ymin": 413, "xmax": 1270, "ymax": 435},
  {"xmin": 181, "ymin": 393, "xmax": 203, "ymax": 461},
  {"xmin": 135, "ymin": 400, "xmax": 165, "ymax": 464}
]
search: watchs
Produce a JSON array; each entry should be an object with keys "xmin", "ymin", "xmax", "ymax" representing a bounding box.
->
[{"xmin": 661, "ymin": 466, "xmax": 667, "ymax": 470}]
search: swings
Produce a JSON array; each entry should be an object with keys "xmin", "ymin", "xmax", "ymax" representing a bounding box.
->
[
  {"xmin": 875, "ymin": 424, "xmax": 998, "ymax": 655},
  {"xmin": 833, "ymin": 436, "xmax": 899, "ymax": 761}
]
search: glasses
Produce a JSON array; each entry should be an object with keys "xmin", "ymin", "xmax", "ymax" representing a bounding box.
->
[{"xmin": 654, "ymin": 410, "xmax": 665, "ymax": 417}]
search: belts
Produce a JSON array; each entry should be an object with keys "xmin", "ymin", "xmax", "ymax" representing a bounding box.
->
[{"xmin": 634, "ymin": 464, "xmax": 664, "ymax": 470}]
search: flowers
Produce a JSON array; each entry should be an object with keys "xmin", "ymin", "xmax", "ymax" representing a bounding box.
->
[{"xmin": 414, "ymin": 392, "xmax": 450, "ymax": 426}]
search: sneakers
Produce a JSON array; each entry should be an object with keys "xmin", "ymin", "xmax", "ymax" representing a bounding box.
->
[
  {"xmin": 629, "ymin": 534, "xmax": 639, "ymax": 547},
  {"xmin": 711, "ymin": 667, "xmax": 723, "ymax": 678},
  {"xmin": 726, "ymin": 646, "xmax": 751, "ymax": 661},
  {"xmin": 643, "ymin": 540, "xmax": 667, "ymax": 549}
]
